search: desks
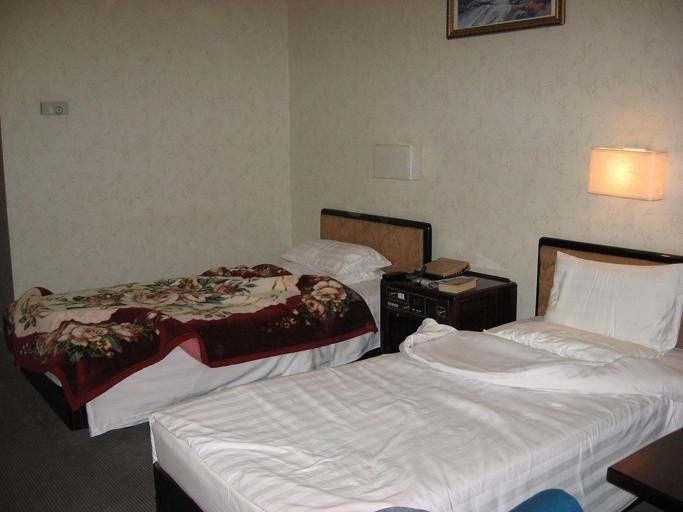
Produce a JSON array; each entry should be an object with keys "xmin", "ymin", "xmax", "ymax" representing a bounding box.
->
[{"xmin": 606, "ymin": 424, "xmax": 680, "ymax": 510}]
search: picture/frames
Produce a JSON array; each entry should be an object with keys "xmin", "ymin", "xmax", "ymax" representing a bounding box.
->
[{"xmin": 442, "ymin": 0, "xmax": 565, "ymax": 39}]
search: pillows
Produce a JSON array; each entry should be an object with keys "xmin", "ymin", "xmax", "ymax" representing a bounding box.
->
[
  {"xmin": 542, "ymin": 247, "xmax": 683, "ymax": 354},
  {"xmin": 278, "ymin": 240, "xmax": 390, "ymax": 285}
]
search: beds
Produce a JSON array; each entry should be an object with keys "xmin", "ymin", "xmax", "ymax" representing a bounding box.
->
[
  {"xmin": 146, "ymin": 236, "xmax": 683, "ymax": 510},
  {"xmin": 4, "ymin": 209, "xmax": 437, "ymax": 437}
]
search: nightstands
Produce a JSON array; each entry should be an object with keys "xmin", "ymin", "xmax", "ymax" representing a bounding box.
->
[{"xmin": 374, "ymin": 265, "xmax": 519, "ymax": 360}]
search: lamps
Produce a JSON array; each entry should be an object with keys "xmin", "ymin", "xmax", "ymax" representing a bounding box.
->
[
  {"xmin": 586, "ymin": 143, "xmax": 668, "ymax": 202},
  {"xmin": 370, "ymin": 141, "xmax": 419, "ymax": 183}
]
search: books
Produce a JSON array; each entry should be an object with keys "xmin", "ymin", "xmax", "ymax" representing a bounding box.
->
[{"xmin": 420, "ymin": 256, "xmax": 476, "ymax": 293}]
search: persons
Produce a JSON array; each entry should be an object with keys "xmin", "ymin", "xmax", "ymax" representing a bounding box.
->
[{"xmin": 457, "ymin": 1, "xmax": 547, "ymax": 26}]
